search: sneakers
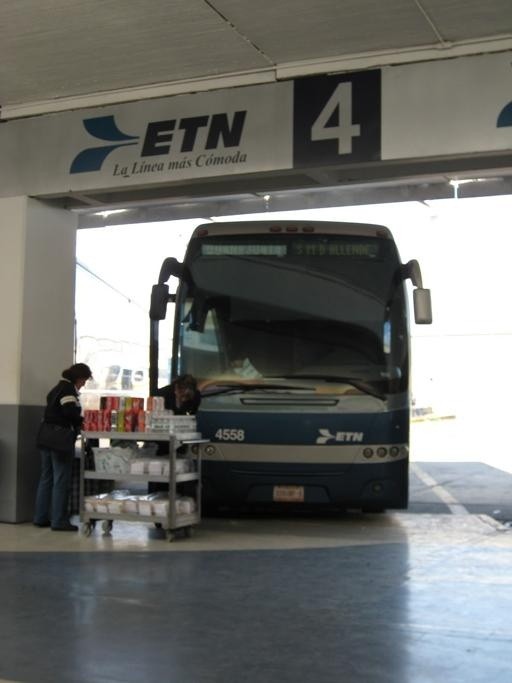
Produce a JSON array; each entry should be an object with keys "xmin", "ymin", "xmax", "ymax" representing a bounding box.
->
[
  {"xmin": 51, "ymin": 525, "xmax": 79, "ymax": 531},
  {"xmin": 33, "ymin": 520, "xmax": 51, "ymax": 527}
]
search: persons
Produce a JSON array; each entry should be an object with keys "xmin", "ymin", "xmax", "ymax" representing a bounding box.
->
[
  {"xmin": 148, "ymin": 371, "xmax": 201, "ymax": 531},
  {"xmin": 33, "ymin": 362, "xmax": 92, "ymax": 531}
]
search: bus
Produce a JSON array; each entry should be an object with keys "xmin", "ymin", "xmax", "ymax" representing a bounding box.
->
[{"xmin": 148, "ymin": 217, "xmax": 431, "ymax": 515}]
